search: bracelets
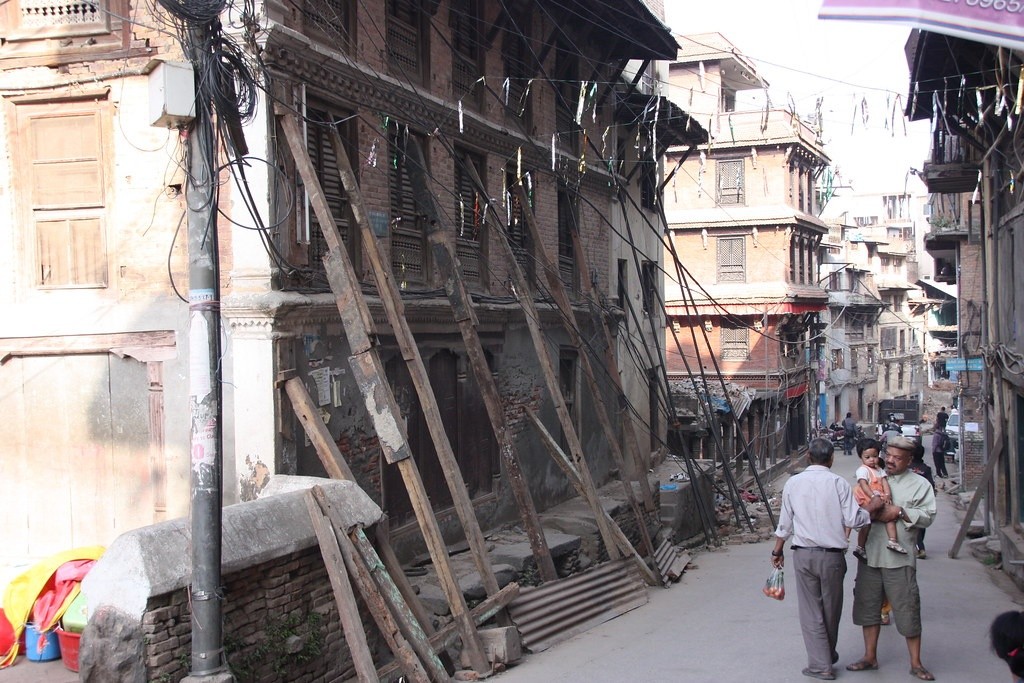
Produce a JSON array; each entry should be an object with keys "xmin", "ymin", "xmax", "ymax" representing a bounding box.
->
[{"xmin": 771, "ymin": 550, "xmax": 784, "ymax": 556}]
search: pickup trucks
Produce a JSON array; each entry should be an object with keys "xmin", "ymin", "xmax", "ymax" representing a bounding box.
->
[{"xmin": 875, "ymin": 399, "xmax": 927, "ymax": 444}]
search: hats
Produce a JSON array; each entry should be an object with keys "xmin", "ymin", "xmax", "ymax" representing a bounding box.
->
[{"xmin": 886, "ymin": 437, "xmax": 915, "ymax": 450}]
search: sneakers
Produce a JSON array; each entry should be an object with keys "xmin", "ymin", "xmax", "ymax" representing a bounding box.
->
[
  {"xmin": 888, "ymin": 542, "xmax": 909, "ymax": 554},
  {"xmin": 852, "ymin": 546, "xmax": 868, "ymax": 564}
]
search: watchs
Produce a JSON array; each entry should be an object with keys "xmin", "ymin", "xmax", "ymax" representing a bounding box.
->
[{"xmin": 899, "ymin": 507, "xmax": 905, "ymax": 519}]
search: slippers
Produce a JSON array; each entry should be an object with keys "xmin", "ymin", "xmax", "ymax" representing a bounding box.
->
[
  {"xmin": 845, "ymin": 658, "xmax": 881, "ymax": 670},
  {"xmin": 910, "ymin": 666, "xmax": 937, "ymax": 681}
]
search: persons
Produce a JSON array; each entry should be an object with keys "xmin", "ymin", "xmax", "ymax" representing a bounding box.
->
[
  {"xmin": 985, "ymin": 609, "xmax": 1024, "ymax": 683},
  {"xmin": 851, "ymin": 438, "xmax": 909, "ymax": 562},
  {"xmin": 916, "ymin": 414, "xmax": 929, "ymax": 432},
  {"xmin": 841, "ymin": 410, "xmax": 857, "ymax": 456},
  {"xmin": 846, "ymin": 435, "xmax": 938, "ymax": 681},
  {"xmin": 770, "ymin": 439, "xmax": 857, "ymax": 680},
  {"xmin": 872, "ymin": 413, "xmax": 908, "ymax": 441},
  {"xmin": 910, "ymin": 443, "xmax": 936, "ymax": 559},
  {"xmin": 931, "ymin": 422, "xmax": 951, "ymax": 479},
  {"xmin": 937, "ymin": 406, "xmax": 949, "ymax": 435}
]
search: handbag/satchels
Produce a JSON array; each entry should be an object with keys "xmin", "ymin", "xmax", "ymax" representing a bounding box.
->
[{"xmin": 763, "ymin": 563, "xmax": 786, "ymax": 600}]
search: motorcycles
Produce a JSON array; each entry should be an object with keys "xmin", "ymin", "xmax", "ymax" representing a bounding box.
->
[{"xmin": 819, "ymin": 423, "xmax": 867, "ymax": 450}]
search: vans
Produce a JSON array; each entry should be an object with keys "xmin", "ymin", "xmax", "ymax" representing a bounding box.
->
[{"xmin": 945, "ymin": 409, "xmax": 959, "ymax": 441}]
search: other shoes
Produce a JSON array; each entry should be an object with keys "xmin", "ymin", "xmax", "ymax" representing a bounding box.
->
[
  {"xmin": 916, "ymin": 550, "xmax": 926, "ymax": 559},
  {"xmin": 830, "ymin": 651, "xmax": 839, "ymax": 665},
  {"xmin": 802, "ymin": 668, "xmax": 836, "ymax": 680}
]
